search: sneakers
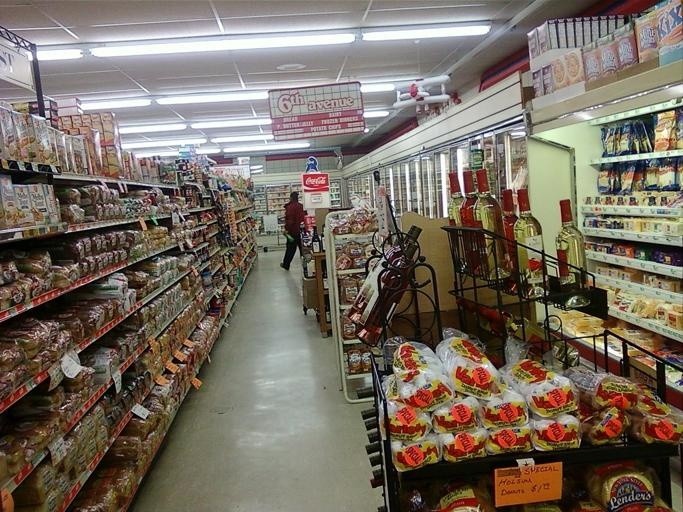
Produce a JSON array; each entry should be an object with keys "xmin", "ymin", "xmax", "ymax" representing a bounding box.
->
[{"xmin": 280, "ymin": 263, "xmax": 290, "ymax": 271}]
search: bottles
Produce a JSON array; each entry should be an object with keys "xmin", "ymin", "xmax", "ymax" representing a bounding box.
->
[
  {"xmin": 350, "ymin": 223, "xmax": 429, "ymax": 346},
  {"xmin": 311, "ymin": 224, "xmax": 326, "ymax": 254},
  {"xmin": 557, "ymin": 198, "xmax": 589, "ymax": 309},
  {"xmin": 447, "ymin": 167, "xmax": 550, "ymax": 301}
]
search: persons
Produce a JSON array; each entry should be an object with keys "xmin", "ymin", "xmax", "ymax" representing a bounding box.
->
[{"xmin": 279, "ymin": 192, "xmax": 305, "ymax": 271}]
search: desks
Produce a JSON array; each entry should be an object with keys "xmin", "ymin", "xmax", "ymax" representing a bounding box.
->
[{"xmin": 297, "ymin": 212, "xmax": 332, "ymax": 336}]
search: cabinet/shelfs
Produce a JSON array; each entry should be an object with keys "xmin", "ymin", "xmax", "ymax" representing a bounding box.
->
[
  {"xmin": 251, "ymin": 179, "xmax": 342, "ymax": 248},
  {"xmin": 359, "ymin": 340, "xmax": 681, "ymax": 506},
  {"xmin": 325, "ymin": 202, "xmax": 386, "ymax": 403},
  {"xmin": 344, "ymin": 116, "xmax": 525, "ymax": 221},
  {"xmin": 0, "ymin": 0, "xmax": 260, "ymax": 512},
  {"xmin": 525, "ymin": 58, "xmax": 682, "ymax": 408}
]
